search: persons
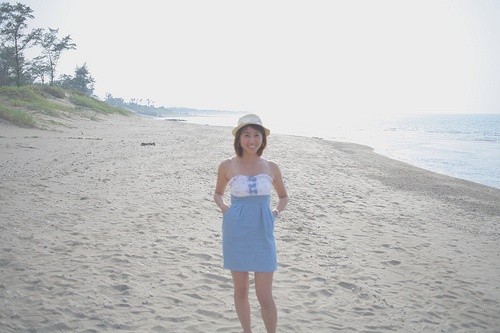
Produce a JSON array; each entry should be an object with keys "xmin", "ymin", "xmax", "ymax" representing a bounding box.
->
[{"xmin": 213, "ymin": 114, "xmax": 288, "ymax": 333}]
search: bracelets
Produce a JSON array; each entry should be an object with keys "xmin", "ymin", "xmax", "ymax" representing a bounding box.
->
[{"xmin": 274, "ymin": 209, "xmax": 279, "ymax": 216}]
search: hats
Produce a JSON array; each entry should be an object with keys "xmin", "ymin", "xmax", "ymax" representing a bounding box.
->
[{"xmin": 231, "ymin": 114, "xmax": 271, "ymax": 136}]
